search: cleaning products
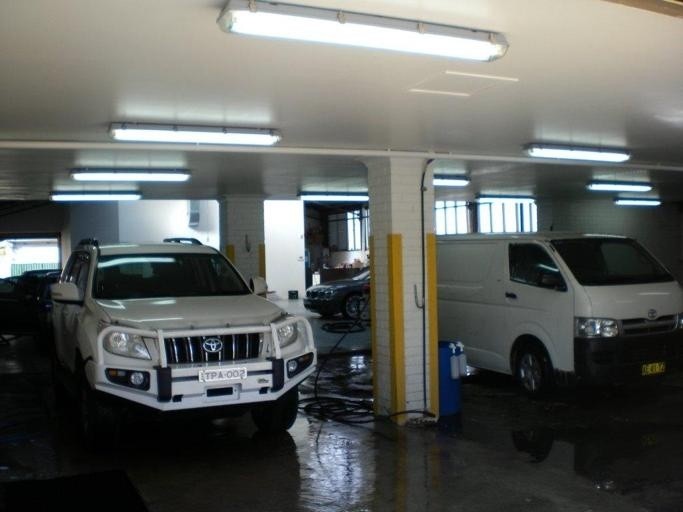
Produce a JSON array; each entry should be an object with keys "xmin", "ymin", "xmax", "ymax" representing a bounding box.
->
[
  {"xmin": 448, "ymin": 343, "xmax": 460, "ymax": 380},
  {"xmin": 455, "ymin": 341, "xmax": 467, "ymax": 377}
]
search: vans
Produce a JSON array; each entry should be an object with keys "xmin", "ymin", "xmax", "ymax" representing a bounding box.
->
[{"xmin": 438, "ymin": 232, "xmax": 681, "ymax": 398}]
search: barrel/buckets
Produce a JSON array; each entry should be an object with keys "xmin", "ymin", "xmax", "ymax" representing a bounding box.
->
[{"xmin": 439, "ymin": 338, "xmax": 464, "ymax": 416}]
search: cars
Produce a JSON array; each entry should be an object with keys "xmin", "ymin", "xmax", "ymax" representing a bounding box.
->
[
  {"xmin": 1, "ymin": 270, "xmax": 63, "ymax": 333},
  {"xmin": 304, "ymin": 269, "xmax": 373, "ymax": 320}
]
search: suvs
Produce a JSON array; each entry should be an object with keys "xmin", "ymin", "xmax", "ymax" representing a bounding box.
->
[{"xmin": 49, "ymin": 236, "xmax": 317, "ymax": 441}]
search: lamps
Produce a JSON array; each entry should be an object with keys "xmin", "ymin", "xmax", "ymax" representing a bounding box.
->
[
  {"xmin": 107, "ymin": 121, "xmax": 282, "ymax": 147},
  {"xmin": 614, "ymin": 199, "xmax": 661, "ymax": 206},
  {"xmin": 297, "ymin": 191, "xmax": 369, "ymax": 202},
  {"xmin": 215, "ymin": 0, "xmax": 508, "ymax": 63},
  {"xmin": 474, "ymin": 194, "xmax": 534, "ymax": 202},
  {"xmin": 48, "ymin": 193, "xmax": 142, "ymax": 203},
  {"xmin": 68, "ymin": 167, "xmax": 192, "ymax": 183},
  {"xmin": 434, "ymin": 175, "xmax": 470, "ymax": 187},
  {"xmin": 585, "ymin": 183, "xmax": 652, "ymax": 192},
  {"xmin": 528, "ymin": 144, "xmax": 630, "ymax": 162}
]
